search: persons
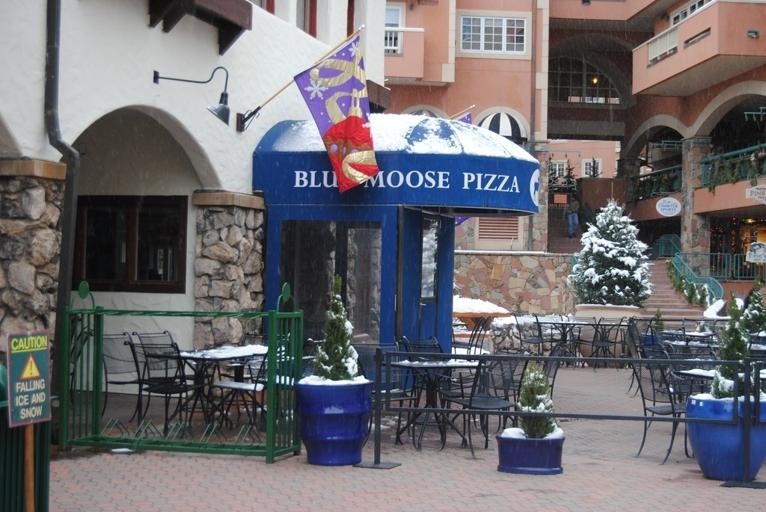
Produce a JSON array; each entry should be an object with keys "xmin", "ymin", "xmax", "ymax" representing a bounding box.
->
[{"xmin": 565, "ymin": 195, "xmax": 580, "ymax": 239}]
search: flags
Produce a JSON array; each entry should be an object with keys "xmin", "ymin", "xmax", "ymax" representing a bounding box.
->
[
  {"xmin": 451, "ymin": 111, "xmax": 473, "ymax": 227},
  {"xmin": 293, "ymin": 31, "xmax": 380, "ymax": 193}
]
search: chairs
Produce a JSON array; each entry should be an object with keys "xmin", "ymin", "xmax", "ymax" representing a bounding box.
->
[
  {"xmin": 517, "ymin": 354, "xmax": 560, "ymax": 417},
  {"xmin": 473, "ymin": 316, "xmax": 495, "ymax": 355},
  {"xmin": 452, "ymin": 317, "xmax": 484, "ymax": 354},
  {"xmin": 627, "ymin": 319, "xmax": 654, "ymax": 358},
  {"xmin": 213, "ymin": 350, "xmax": 271, "ymax": 429},
  {"xmin": 633, "ymin": 364, "xmax": 692, "ymax": 464},
  {"xmin": 514, "ymin": 311, "xmax": 555, "ymax": 356},
  {"xmin": 621, "ymin": 330, "xmax": 669, "ymax": 396},
  {"xmin": 396, "ymin": 336, "xmax": 452, "ymax": 448},
  {"xmin": 125, "ymin": 341, "xmax": 206, "ymax": 436},
  {"xmin": 470, "ymin": 353, "xmax": 519, "ymax": 424},
  {"xmin": 443, "ymin": 355, "xmax": 516, "ymax": 444},
  {"xmin": 134, "ymin": 329, "xmax": 212, "ymax": 380},
  {"xmin": 516, "ymin": 344, "xmax": 564, "ymax": 402}
]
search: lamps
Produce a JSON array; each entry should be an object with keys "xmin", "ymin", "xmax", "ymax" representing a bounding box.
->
[{"xmin": 149, "ymin": 66, "xmax": 231, "ymax": 126}]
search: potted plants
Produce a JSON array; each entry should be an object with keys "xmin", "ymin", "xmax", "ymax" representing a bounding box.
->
[
  {"xmin": 495, "ymin": 363, "xmax": 565, "ymax": 474},
  {"xmin": 294, "ymin": 274, "xmax": 372, "ymax": 466},
  {"xmin": 567, "ymin": 199, "xmax": 654, "ymax": 369},
  {"xmin": 686, "ymin": 278, "xmax": 766, "ymax": 481}
]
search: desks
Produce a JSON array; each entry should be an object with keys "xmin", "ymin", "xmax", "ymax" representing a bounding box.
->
[
  {"xmin": 533, "ymin": 321, "xmax": 589, "ymax": 366},
  {"xmin": 578, "ymin": 322, "xmax": 631, "ymax": 368},
  {"xmin": 147, "ymin": 344, "xmax": 268, "ymax": 428},
  {"xmin": 382, "ymin": 358, "xmax": 485, "ymax": 447}
]
